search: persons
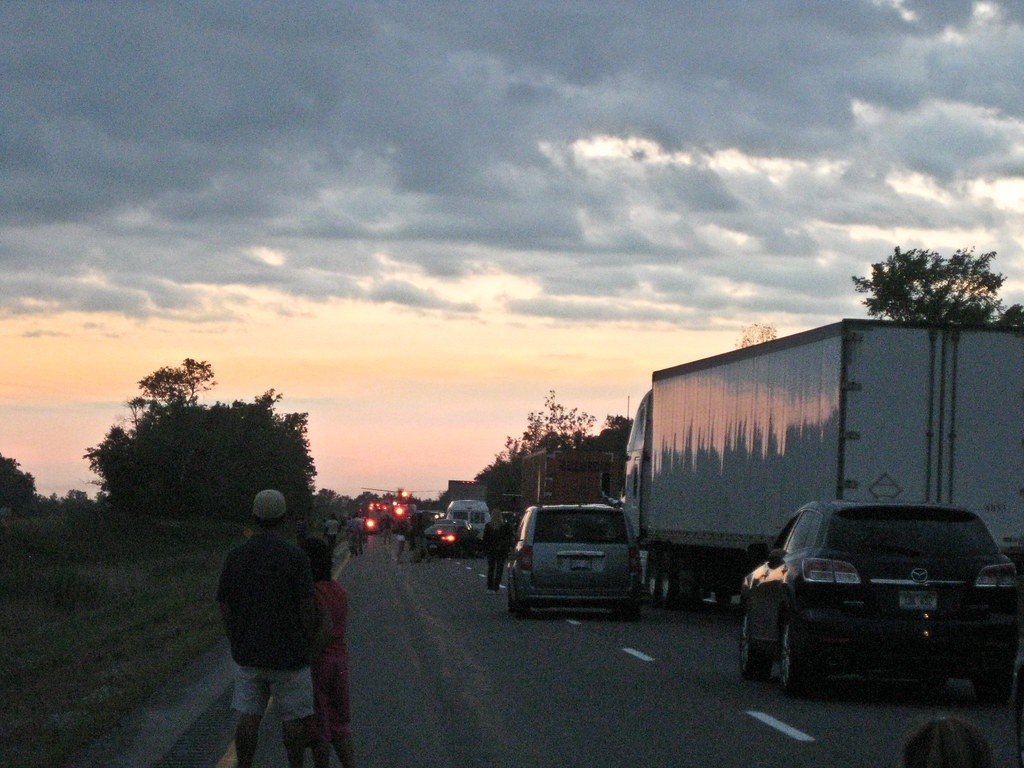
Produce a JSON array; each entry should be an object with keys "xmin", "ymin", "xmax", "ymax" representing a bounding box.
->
[
  {"xmin": 216, "ymin": 488, "xmax": 318, "ymax": 768},
  {"xmin": 480, "ymin": 508, "xmax": 515, "ymax": 596},
  {"xmin": 299, "ymin": 537, "xmax": 356, "ymax": 768},
  {"xmin": 901, "ymin": 715, "xmax": 992, "ymax": 768},
  {"xmin": 288, "ymin": 507, "xmax": 432, "ymax": 585}
]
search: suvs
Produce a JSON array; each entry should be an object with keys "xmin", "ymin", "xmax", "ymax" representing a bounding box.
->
[{"xmin": 739, "ymin": 501, "xmax": 1020, "ymax": 705}]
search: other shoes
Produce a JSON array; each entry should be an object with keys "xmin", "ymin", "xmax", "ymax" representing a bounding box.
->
[{"xmin": 487, "ymin": 588, "xmax": 504, "ymax": 595}]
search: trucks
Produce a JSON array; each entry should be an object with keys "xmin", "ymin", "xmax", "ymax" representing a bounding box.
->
[
  {"xmin": 517, "ymin": 448, "xmax": 622, "ymax": 501},
  {"xmin": 623, "ymin": 318, "xmax": 1024, "ymax": 609}
]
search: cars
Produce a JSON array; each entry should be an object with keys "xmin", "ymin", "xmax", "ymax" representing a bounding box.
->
[
  {"xmin": 412, "ymin": 510, "xmax": 444, "ymax": 519},
  {"xmin": 424, "ymin": 520, "xmax": 478, "ymax": 555}
]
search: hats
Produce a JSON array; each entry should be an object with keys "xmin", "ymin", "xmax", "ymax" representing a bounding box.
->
[{"xmin": 253, "ymin": 489, "xmax": 286, "ymax": 521}]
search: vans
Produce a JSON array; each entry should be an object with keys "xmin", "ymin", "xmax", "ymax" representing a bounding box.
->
[
  {"xmin": 506, "ymin": 503, "xmax": 646, "ymax": 622},
  {"xmin": 447, "ymin": 500, "xmax": 491, "ymax": 542}
]
